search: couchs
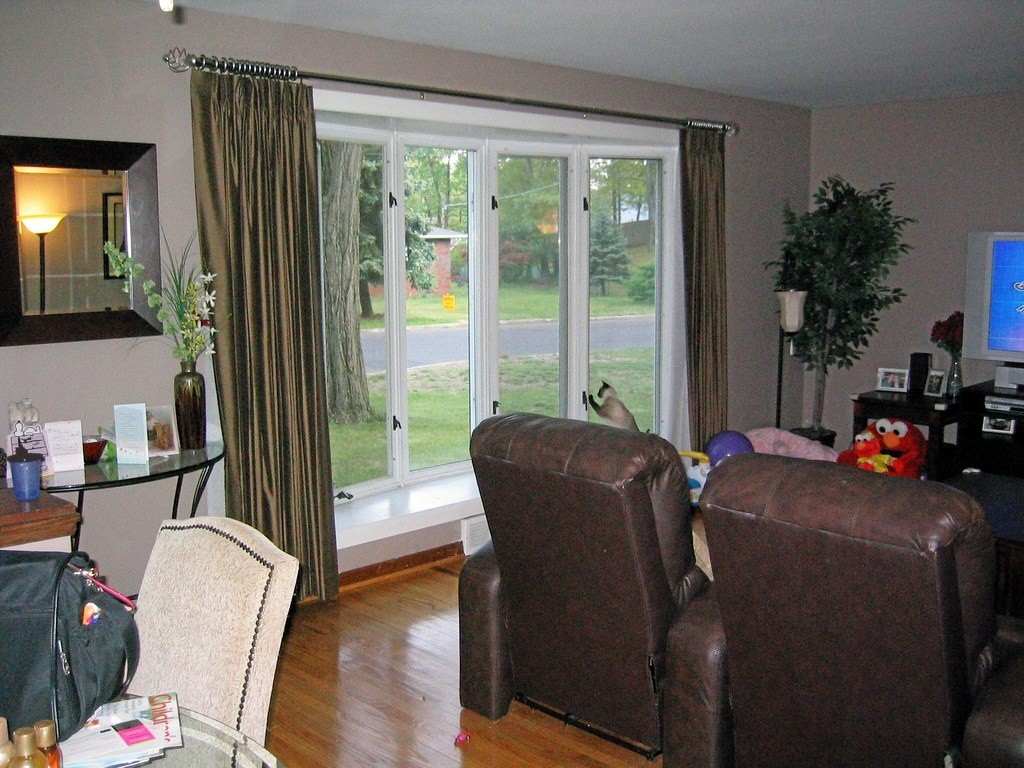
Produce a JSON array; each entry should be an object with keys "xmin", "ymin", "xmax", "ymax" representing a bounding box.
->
[{"xmin": 456, "ymin": 414, "xmax": 1024, "ymax": 768}]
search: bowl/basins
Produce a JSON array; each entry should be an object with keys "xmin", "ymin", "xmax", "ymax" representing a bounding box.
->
[{"xmin": 83, "ymin": 437, "xmax": 107, "ymax": 466}]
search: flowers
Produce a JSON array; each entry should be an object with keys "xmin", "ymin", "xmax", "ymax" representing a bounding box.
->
[
  {"xmin": 120, "ymin": 229, "xmax": 235, "ymax": 363},
  {"xmin": 931, "ymin": 311, "xmax": 966, "ymax": 354}
]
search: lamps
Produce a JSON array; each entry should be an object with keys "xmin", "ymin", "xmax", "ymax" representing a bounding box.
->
[
  {"xmin": 17, "ymin": 210, "xmax": 69, "ymax": 316},
  {"xmin": 775, "ymin": 288, "xmax": 810, "ymax": 429}
]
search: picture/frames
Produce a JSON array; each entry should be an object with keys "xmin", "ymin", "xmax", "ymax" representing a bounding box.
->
[
  {"xmin": 99, "ymin": 191, "xmax": 124, "ymax": 278},
  {"xmin": 875, "ymin": 365, "xmax": 908, "ymax": 392},
  {"xmin": 924, "ymin": 368, "xmax": 946, "ymax": 397}
]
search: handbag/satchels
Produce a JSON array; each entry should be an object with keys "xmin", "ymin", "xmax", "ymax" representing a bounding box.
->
[{"xmin": 0, "ymin": 550, "xmax": 140, "ymax": 742}]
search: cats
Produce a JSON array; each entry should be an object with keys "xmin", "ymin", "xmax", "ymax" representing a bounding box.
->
[{"xmin": 588, "ymin": 381, "xmax": 650, "ymax": 434}]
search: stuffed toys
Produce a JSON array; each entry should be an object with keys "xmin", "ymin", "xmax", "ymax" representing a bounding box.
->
[{"xmin": 836, "ymin": 417, "xmax": 927, "ymax": 479}]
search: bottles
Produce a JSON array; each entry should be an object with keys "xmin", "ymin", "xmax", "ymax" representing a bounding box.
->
[
  {"xmin": 33, "ymin": 720, "xmax": 63, "ymax": 768},
  {"xmin": 0, "ymin": 717, "xmax": 13, "ymax": 768},
  {"xmin": 8, "ymin": 728, "xmax": 48, "ymax": 768}
]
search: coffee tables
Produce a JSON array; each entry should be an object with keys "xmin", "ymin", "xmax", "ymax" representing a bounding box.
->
[{"xmin": 949, "ymin": 476, "xmax": 1022, "ymax": 644}]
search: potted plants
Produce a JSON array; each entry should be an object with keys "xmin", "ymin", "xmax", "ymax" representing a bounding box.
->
[{"xmin": 772, "ymin": 175, "xmax": 921, "ymax": 448}]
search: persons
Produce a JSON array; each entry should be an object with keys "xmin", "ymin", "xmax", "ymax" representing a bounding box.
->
[
  {"xmin": 881, "ymin": 374, "xmax": 904, "ymax": 389},
  {"xmin": 927, "ymin": 375, "xmax": 942, "ymax": 394}
]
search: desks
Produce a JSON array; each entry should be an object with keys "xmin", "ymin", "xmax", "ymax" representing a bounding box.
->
[
  {"xmin": 109, "ymin": 693, "xmax": 289, "ymax": 767},
  {"xmin": 951, "ymin": 381, "xmax": 1024, "ymax": 475},
  {"xmin": 45, "ymin": 439, "xmax": 227, "ymax": 552},
  {"xmin": 0, "ymin": 489, "xmax": 87, "ymax": 558},
  {"xmin": 850, "ymin": 389, "xmax": 966, "ymax": 471}
]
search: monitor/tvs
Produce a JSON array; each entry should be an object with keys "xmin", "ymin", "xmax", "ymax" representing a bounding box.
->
[{"xmin": 980, "ymin": 235, "xmax": 1024, "ymax": 363}]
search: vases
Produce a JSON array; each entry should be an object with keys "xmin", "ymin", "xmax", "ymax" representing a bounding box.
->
[
  {"xmin": 944, "ymin": 354, "xmax": 962, "ymax": 397},
  {"xmin": 172, "ymin": 361, "xmax": 204, "ymax": 447}
]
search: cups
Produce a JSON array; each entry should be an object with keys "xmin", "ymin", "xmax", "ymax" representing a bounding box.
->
[{"xmin": 8, "ymin": 447, "xmax": 45, "ymax": 501}]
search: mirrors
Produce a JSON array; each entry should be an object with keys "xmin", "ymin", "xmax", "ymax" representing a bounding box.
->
[{"xmin": 0, "ymin": 133, "xmax": 163, "ymax": 346}]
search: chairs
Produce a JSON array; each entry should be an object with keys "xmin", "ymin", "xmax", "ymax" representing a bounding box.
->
[{"xmin": 125, "ymin": 516, "xmax": 304, "ymax": 767}]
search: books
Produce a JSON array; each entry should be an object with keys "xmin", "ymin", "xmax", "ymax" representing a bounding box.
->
[{"xmin": 59, "ymin": 692, "xmax": 183, "ymax": 768}]
